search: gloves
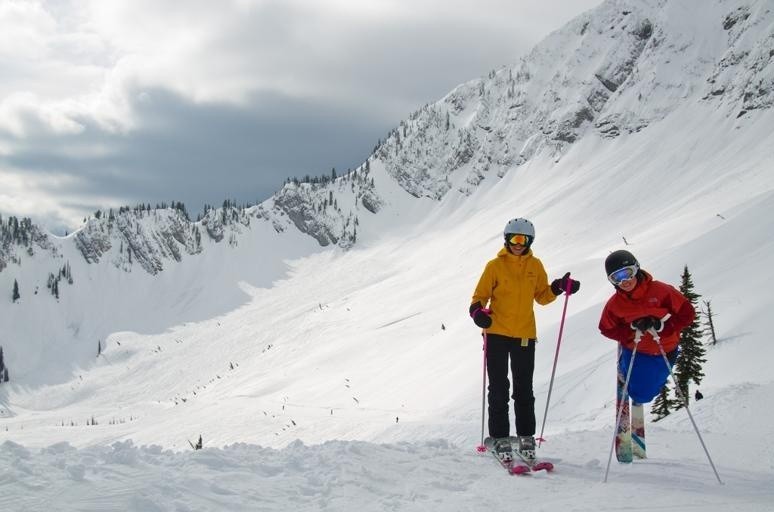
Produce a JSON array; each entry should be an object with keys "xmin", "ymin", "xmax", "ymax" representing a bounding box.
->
[
  {"xmin": 551, "ymin": 271, "xmax": 580, "ymax": 296},
  {"xmin": 468, "ymin": 301, "xmax": 493, "ymax": 329},
  {"xmin": 631, "ymin": 315, "xmax": 661, "ymax": 330}
]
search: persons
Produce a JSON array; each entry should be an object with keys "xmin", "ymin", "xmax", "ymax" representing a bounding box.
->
[
  {"xmin": 598, "ymin": 249, "xmax": 697, "ymax": 403},
  {"xmin": 468, "ymin": 217, "xmax": 581, "ymax": 465}
]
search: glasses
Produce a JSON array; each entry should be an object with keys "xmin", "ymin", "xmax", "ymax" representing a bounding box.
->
[
  {"xmin": 506, "ymin": 234, "xmax": 532, "ymax": 246},
  {"xmin": 607, "ymin": 266, "xmax": 637, "ymax": 285}
]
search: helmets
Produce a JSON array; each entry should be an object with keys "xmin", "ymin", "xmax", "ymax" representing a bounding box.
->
[
  {"xmin": 605, "ymin": 250, "xmax": 640, "ymax": 286},
  {"xmin": 503, "ymin": 217, "xmax": 535, "ymax": 241}
]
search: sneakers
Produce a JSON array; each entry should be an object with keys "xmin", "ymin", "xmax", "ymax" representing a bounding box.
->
[
  {"xmin": 492, "ymin": 437, "xmax": 512, "ymax": 458},
  {"xmin": 518, "ymin": 435, "xmax": 536, "ymax": 456}
]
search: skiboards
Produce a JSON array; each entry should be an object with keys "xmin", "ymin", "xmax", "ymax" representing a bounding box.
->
[
  {"xmin": 616, "ymin": 336, "xmax": 646, "ymax": 464},
  {"xmin": 483, "ymin": 435, "xmax": 553, "ymax": 476}
]
